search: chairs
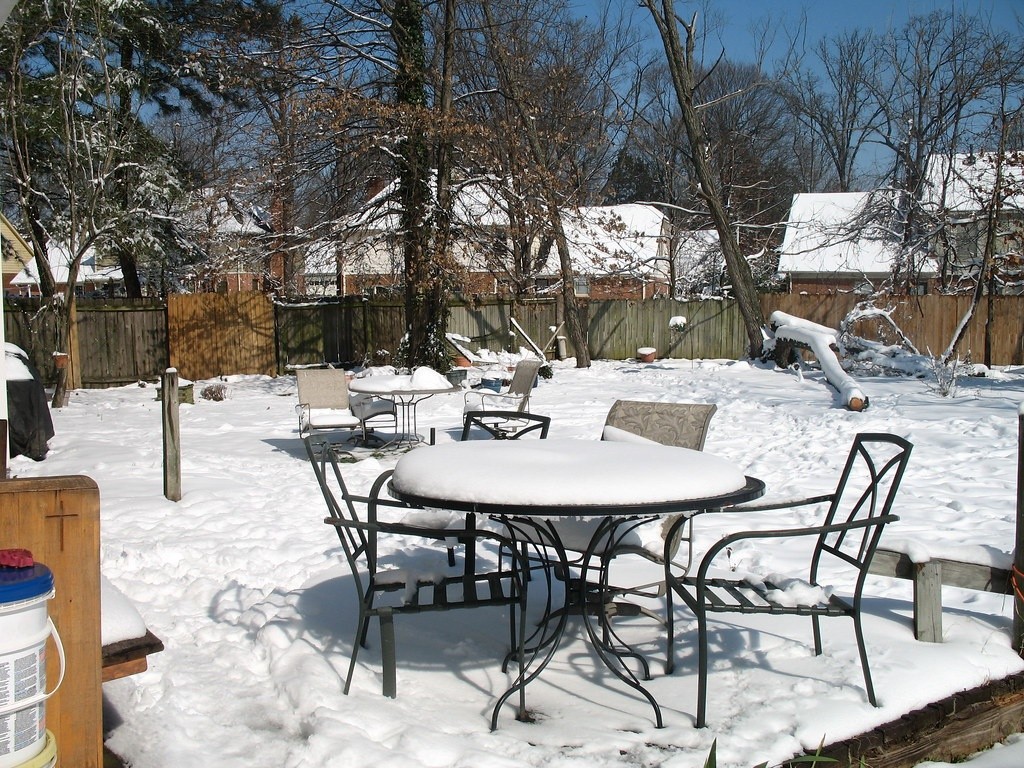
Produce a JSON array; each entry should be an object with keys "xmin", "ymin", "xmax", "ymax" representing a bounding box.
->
[
  {"xmin": 443, "ymin": 411, "xmax": 551, "ymax": 569},
  {"xmin": 301, "ymin": 432, "xmax": 534, "ymax": 726},
  {"xmin": 348, "ymin": 394, "xmax": 398, "ymax": 446},
  {"xmin": 662, "ymin": 431, "xmax": 914, "ymax": 726},
  {"xmin": 501, "ymin": 399, "xmax": 722, "ymax": 680},
  {"xmin": 295, "ymin": 368, "xmax": 361, "ymax": 462},
  {"xmin": 463, "ymin": 359, "xmax": 542, "ymax": 434}
]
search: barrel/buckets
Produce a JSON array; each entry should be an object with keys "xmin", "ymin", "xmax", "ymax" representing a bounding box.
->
[{"xmin": 0, "ymin": 561, "xmax": 66, "ymax": 767}]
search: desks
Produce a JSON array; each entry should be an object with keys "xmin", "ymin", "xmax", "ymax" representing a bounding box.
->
[
  {"xmin": 384, "ymin": 475, "xmax": 765, "ymax": 732},
  {"xmin": 348, "ymin": 383, "xmax": 463, "ymax": 456}
]
